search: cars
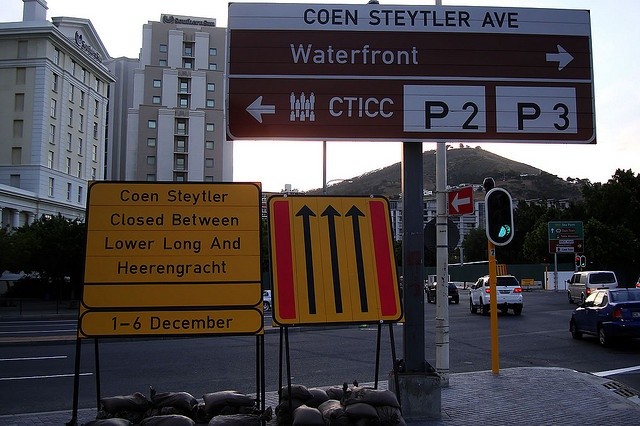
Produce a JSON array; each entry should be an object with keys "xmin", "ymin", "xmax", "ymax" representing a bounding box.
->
[
  {"xmin": 570, "ymin": 288, "xmax": 639, "ymax": 345},
  {"xmin": 429, "ymin": 282, "xmax": 458, "ymax": 303}
]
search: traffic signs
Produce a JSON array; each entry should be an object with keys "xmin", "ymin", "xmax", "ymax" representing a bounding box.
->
[
  {"xmin": 548, "ymin": 222, "xmax": 584, "ymax": 253},
  {"xmin": 225, "ymin": 1, "xmax": 596, "ymax": 145}
]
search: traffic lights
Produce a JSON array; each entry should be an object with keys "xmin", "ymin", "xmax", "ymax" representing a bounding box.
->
[
  {"xmin": 576, "ymin": 255, "xmax": 580, "ymax": 268},
  {"xmin": 485, "ymin": 186, "xmax": 512, "ymax": 244},
  {"xmin": 582, "ymin": 256, "xmax": 585, "ymax": 268}
]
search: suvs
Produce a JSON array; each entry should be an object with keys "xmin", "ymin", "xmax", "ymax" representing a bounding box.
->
[{"xmin": 469, "ymin": 276, "xmax": 523, "ymax": 315}]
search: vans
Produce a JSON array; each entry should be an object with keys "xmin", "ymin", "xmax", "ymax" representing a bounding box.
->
[{"xmin": 567, "ymin": 272, "xmax": 618, "ymax": 303}]
